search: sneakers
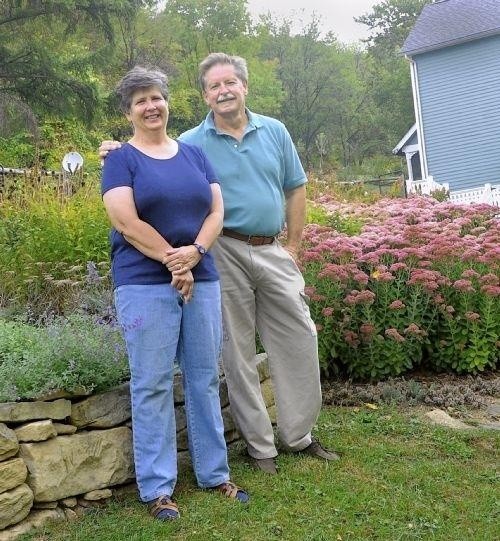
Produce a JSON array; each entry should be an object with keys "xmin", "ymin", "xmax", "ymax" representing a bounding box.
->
[
  {"xmin": 250, "ymin": 457, "xmax": 277, "ymax": 476},
  {"xmin": 278, "ymin": 437, "xmax": 345, "ymax": 461}
]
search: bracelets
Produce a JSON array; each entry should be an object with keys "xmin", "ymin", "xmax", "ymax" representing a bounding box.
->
[{"xmin": 193, "ymin": 242, "xmax": 207, "ymax": 255}]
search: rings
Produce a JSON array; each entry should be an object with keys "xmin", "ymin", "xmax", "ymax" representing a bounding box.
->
[{"xmin": 179, "ymin": 263, "xmax": 183, "ymax": 269}]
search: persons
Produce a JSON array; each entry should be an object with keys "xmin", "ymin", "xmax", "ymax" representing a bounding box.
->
[
  {"xmin": 98, "ymin": 52, "xmax": 342, "ymax": 476},
  {"xmin": 100, "ymin": 65, "xmax": 251, "ymax": 521}
]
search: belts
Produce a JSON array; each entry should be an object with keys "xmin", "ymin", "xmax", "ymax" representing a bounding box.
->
[{"xmin": 223, "ymin": 227, "xmax": 274, "ymax": 245}]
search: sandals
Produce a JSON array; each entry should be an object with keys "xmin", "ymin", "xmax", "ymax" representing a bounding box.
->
[
  {"xmin": 147, "ymin": 495, "xmax": 181, "ymax": 519},
  {"xmin": 216, "ymin": 481, "xmax": 247, "ymax": 498}
]
